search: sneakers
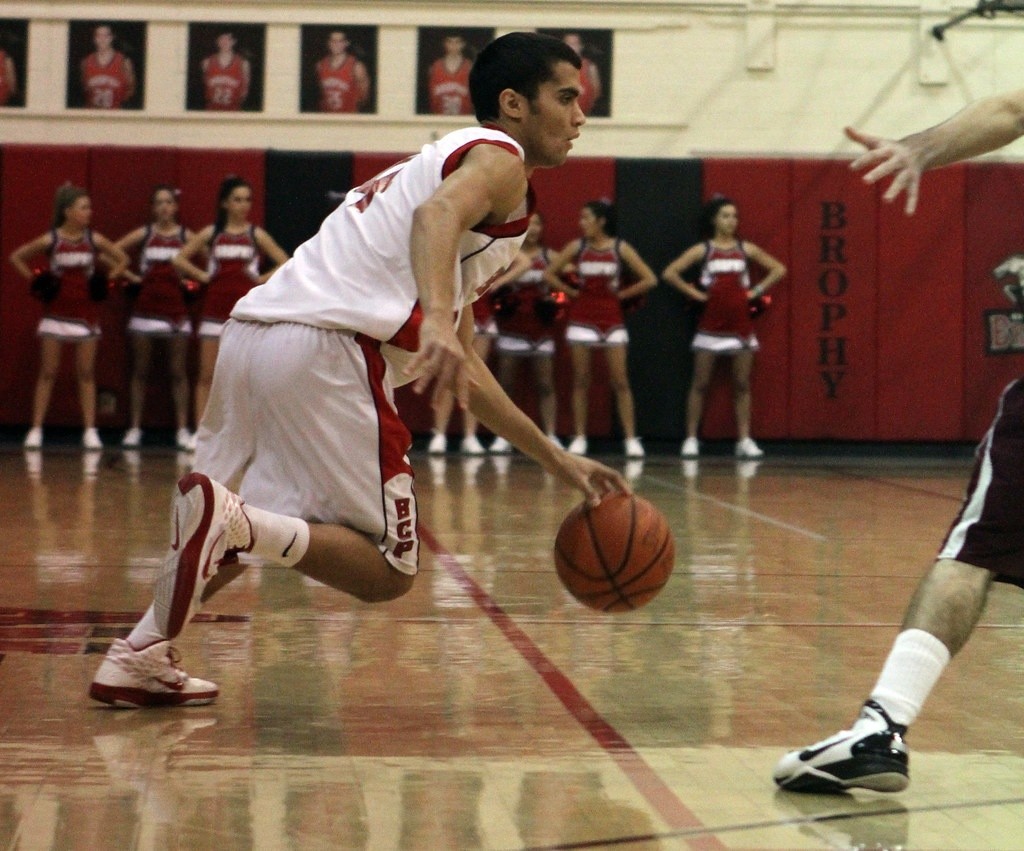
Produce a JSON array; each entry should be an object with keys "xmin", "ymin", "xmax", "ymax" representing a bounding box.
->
[
  {"xmin": 90, "ymin": 635, "xmax": 220, "ymax": 704},
  {"xmin": 89, "ymin": 704, "xmax": 218, "ymax": 785},
  {"xmin": 155, "ymin": 473, "xmax": 253, "ymax": 637},
  {"xmin": 775, "ymin": 695, "xmax": 914, "ymax": 791},
  {"xmin": 772, "ymin": 789, "xmax": 909, "ymax": 851}
]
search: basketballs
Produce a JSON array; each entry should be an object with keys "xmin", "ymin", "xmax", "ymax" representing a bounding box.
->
[{"xmin": 552, "ymin": 494, "xmax": 676, "ymax": 613}]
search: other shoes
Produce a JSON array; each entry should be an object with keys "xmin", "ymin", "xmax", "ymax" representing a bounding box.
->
[
  {"xmin": 426, "ymin": 433, "xmax": 447, "ymax": 454},
  {"xmin": 736, "ymin": 439, "xmax": 763, "ymax": 458},
  {"xmin": 461, "ymin": 435, "xmax": 485, "ymax": 453},
  {"xmin": 681, "ymin": 438, "xmax": 699, "ymax": 458},
  {"xmin": 568, "ymin": 438, "xmax": 587, "ymax": 455},
  {"xmin": 174, "ymin": 427, "xmax": 201, "ymax": 451},
  {"xmin": 488, "ymin": 436, "xmax": 513, "ymax": 452},
  {"xmin": 623, "ymin": 436, "xmax": 644, "ymax": 457},
  {"xmin": 124, "ymin": 428, "xmax": 142, "ymax": 447},
  {"xmin": 546, "ymin": 434, "xmax": 566, "ymax": 450},
  {"xmin": 82, "ymin": 428, "xmax": 104, "ymax": 449},
  {"xmin": 26, "ymin": 425, "xmax": 43, "ymax": 448}
]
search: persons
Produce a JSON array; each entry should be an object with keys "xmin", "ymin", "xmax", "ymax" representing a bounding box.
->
[
  {"xmin": 772, "ymin": 86, "xmax": 1024, "ymax": 793},
  {"xmin": 90, "ymin": 30, "xmax": 633, "ymax": 709},
  {"xmin": 562, "ymin": 32, "xmax": 600, "ymax": 115},
  {"xmin": 312, "ymin": 27, "xmax": 369, "ymax": 112},
  {"xmin": 487, "ymin": 208, "xmax": 576, "ymax": 453},
  {"xmin": 427, "ymin": 240, "xmax": 531, "ymax": 455},
  {"xmin": 8, "ymin": 180, "xmax": 131, "ymax": 450},
  {"xmin": 98, "ymin": 181, "xmax": 212, "ymax": 447},
  {"xmin": 0, "ymin": 47, "xmax": 16, "ymax": 104},
  {"xmin": 76, "ymin": 24, "xmax": 135, "ymax": 108},
  {"xmin": 174, "ymin": 177, "xmax": 290, "ymax": 429},
  {"xmin": 543, "ymin": 197, "xmax": 657, "ymax": 458},
  {"xmin": 199, "ymin": 25, "xmax": 249, "ymax": 112},
  {"xmin": 660, "ymin": 195, "xmax": 787, "ymax": 458},
  {"xmin": 426, "ymin": 30, "xmax": 473, "ymax": 114}
]
